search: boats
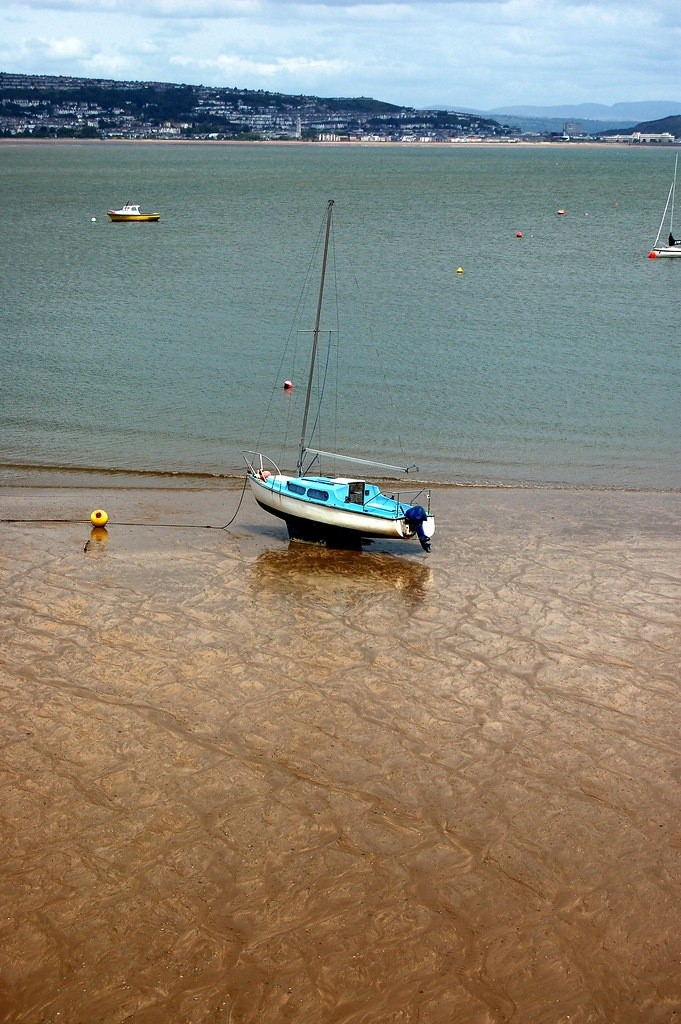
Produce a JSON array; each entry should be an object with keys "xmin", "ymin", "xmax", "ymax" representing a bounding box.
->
[{"xmin": 106, "ymin": 204, "xmax": 161, "ymax": 222}]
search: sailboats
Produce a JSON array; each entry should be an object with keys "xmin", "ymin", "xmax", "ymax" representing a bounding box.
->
[
  {"xmin": 242, "ymin": 198, "xmax": 438, "ymax": 554},
  {"xmin": 649, "ymin": 155, "xmax": 681, "ymax": 259}
]
